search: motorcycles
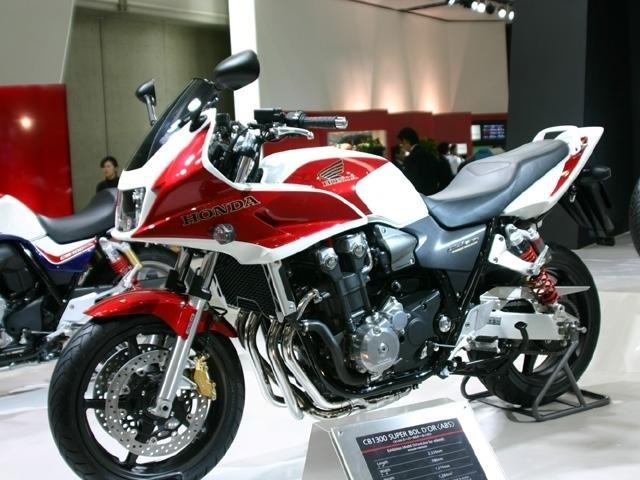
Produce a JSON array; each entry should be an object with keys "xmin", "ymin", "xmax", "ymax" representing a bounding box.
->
[
  {"xmin": 46, "ymin": 49, "xmax": 616, "ymax": 480},
  {"xmin": 0, "ymin": 187, "xmax": 195, "ymax": 372}
]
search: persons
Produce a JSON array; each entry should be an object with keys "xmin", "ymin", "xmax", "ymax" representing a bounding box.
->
[
  {"xmin": 397, "ymin": 127, "xmax": 451, "ymax": 196},
  {"xmin": 391, "ymin": 144, "xmax": 407, "ymax": 171},
  {"xmin": 96, "ymin": 155, "xmax": 120, "ymax": 191},
  {"xmin": 439, "ymin": 142, "xmax": 461, "ymax": 174},
  {"xmin": 450, "ymin": 143, "xmax": 465, "ymax": 162}
]
